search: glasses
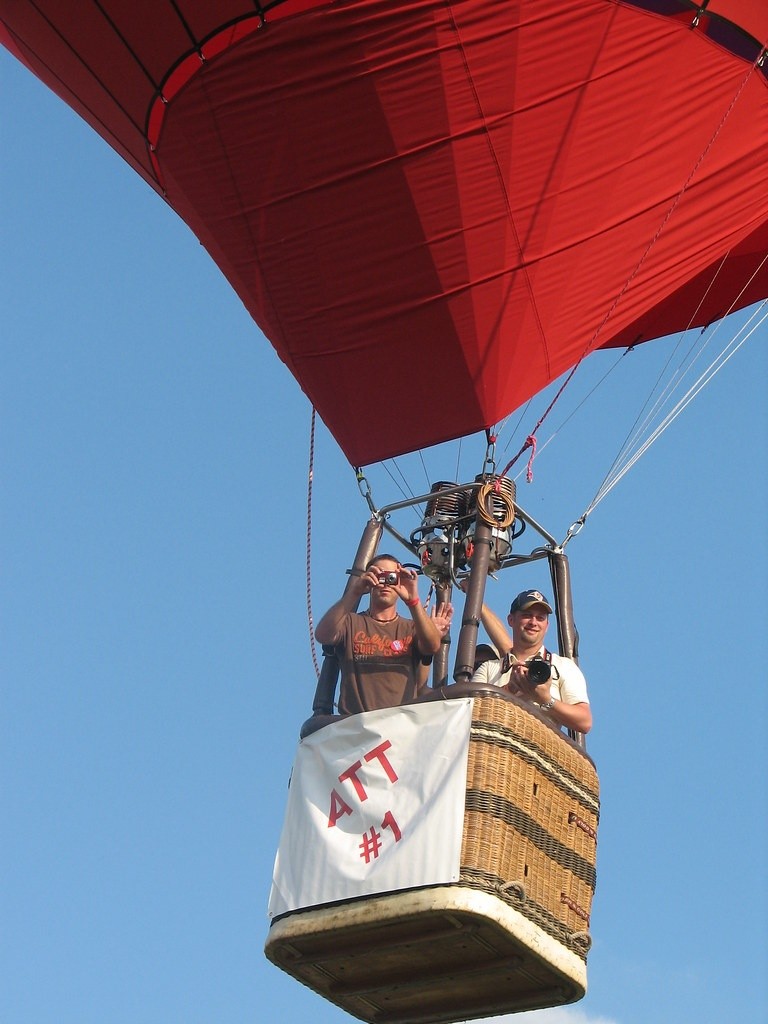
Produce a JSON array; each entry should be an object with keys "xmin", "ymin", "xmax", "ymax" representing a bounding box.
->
[{"xmin": 473, "ymin": 661, "xmax": 486, "ymax": 671}]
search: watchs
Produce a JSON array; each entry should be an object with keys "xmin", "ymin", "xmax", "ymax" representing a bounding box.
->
[{"xmin": 540, "ymin": 697, "xmax": 556, "ymax": 712}]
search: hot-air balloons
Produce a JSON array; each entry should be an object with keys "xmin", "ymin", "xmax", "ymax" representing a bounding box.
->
[{"xmin": 0, "ymin": 0, "xmax": 768, "ymax": 1023}]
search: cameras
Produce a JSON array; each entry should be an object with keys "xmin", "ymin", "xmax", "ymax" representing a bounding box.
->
[
  {"xmin": 377, "ymin": 571, "xmax": 399, "ymax": 586},
  {"xmin": 523, "ymin": 656, "xmax": 551, "ymax": 685}
]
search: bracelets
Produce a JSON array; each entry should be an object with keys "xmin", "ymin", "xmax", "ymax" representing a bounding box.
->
[{"xmin": 405, "ymin": 598, "xmax": 420, "ymax": 607}]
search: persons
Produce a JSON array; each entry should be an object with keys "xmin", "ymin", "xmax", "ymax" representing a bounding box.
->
[
  {"xmin": 312, "ymin": 553, "xmax": 442, "ymax": 715},
  {"xmin": 471, "ymin": 586, "xmax": 591, "ymax": 733},
  {"xmin": 426, "ymin": 577, "xmax": 516, "ymax": 681}
]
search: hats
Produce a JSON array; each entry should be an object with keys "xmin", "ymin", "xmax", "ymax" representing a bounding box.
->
[
  {"xmin": 511, "ymin": 590, "xmax": 552, "ymax": 614},
  {"xmin": 476, "ymin": 643, "xmax": 499, "ymax": 660}
]
